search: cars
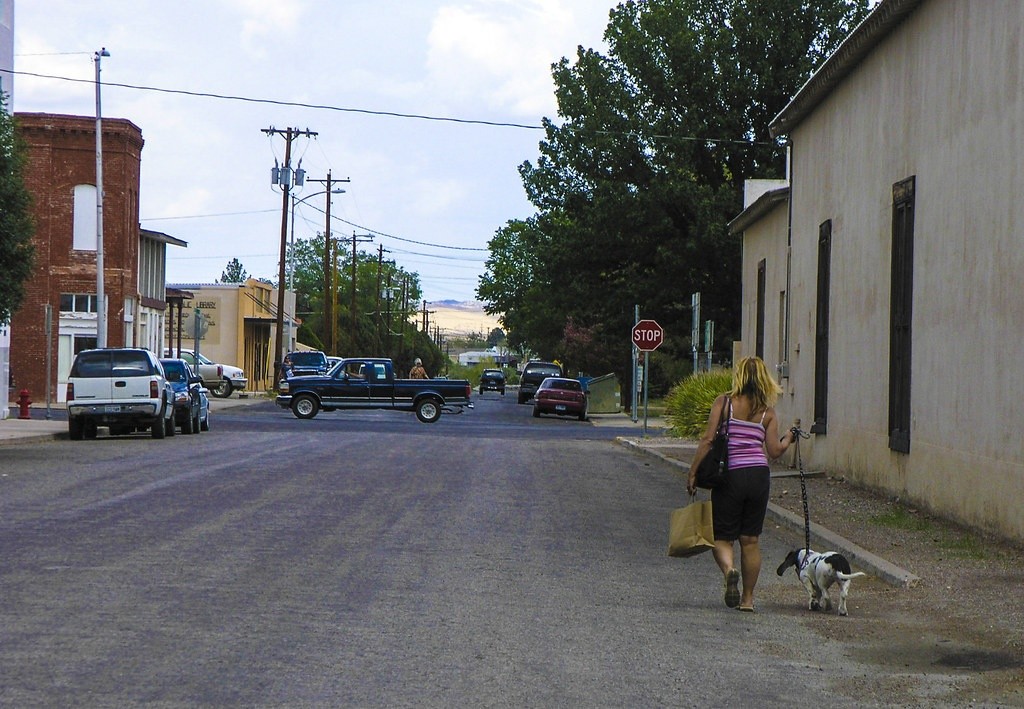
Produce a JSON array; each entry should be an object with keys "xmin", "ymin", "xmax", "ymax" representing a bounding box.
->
[
  {"xmin": 478, "ymin": 368, "xmax": 508, "ymax": 395},
  {"xmin": 163, "ymin": 343, "xmax": 248, "ymax": 398},
  {"xmin": 532, "ymin": 376, "xmax": 592, "ymax": 422},
  {"xmin": 159, "ymin": 357, "xmax": 202, "ymax": 434},
  {"xmin": 280, "ymin": 348, "xmax": 398, "ymax": 380},
  {"xmin": 198, "ymin": 382, "xmax": 211, "ymax": 432}
]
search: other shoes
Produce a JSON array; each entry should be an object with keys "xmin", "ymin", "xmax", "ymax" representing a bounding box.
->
[
  {"xmin": 723, "ymin": 567, "xmax": 740, "ymax": 608},
  {"xmin": 737, "ymin": 599, "xmax": 753, "ymax": 611}
]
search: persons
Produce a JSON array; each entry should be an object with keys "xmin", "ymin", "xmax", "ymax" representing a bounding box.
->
[
  {"xmin": 687, "ymin": 356, "xmax": 799, "ymax": 612},
  {"xmin": 347, "ymin": 370, "xmax": 365, "ymax": 378},
  {"xmin": 279, "ymin": 356, "xmax": 293, "ymax": 378},
  {"xmin": 409, "ymin": 358, "xmax": 429, "ymax": 379}
]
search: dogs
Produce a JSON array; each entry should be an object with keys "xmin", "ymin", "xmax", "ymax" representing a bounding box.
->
[{"xmin": 776, "ymin": 547, "xmax": 866, "ymax": 618}]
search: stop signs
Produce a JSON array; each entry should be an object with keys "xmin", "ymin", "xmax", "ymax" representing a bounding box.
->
[{"xmin": 631, "ymin": 319, "xmax": 664, "ymax": 352}]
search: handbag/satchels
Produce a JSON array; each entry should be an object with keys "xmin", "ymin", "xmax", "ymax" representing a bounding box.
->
[
  {"xmin": 668, "ymin": 490, "xmax": 715, "ymax": 558},
  {"xmin": 694, "ymin": 435, "xmax": 729, "ymax": 490}
]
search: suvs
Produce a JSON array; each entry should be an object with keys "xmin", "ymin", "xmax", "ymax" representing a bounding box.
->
[{"xmin": 518, "ymin": 358, "xmax": 564, "ymax": 405}]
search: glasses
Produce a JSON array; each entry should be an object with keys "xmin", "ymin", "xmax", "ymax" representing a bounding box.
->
[{"xmin": 286, "ymin": 358, "xmax": 290, "ymax": 359}]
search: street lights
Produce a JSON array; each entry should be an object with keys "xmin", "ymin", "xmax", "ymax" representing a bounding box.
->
[{"xmin": 289, "ymin": 185, "xmax": 376, "ymax": 354}]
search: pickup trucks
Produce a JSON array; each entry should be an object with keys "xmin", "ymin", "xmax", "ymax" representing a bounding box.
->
[
  {"xmin": 275, "ymin": 357, "xmax": 474, "ymax": 424},
  {"xmin": 63, "ymin": 346, "xmax": 177, "ymax": 439}
]
